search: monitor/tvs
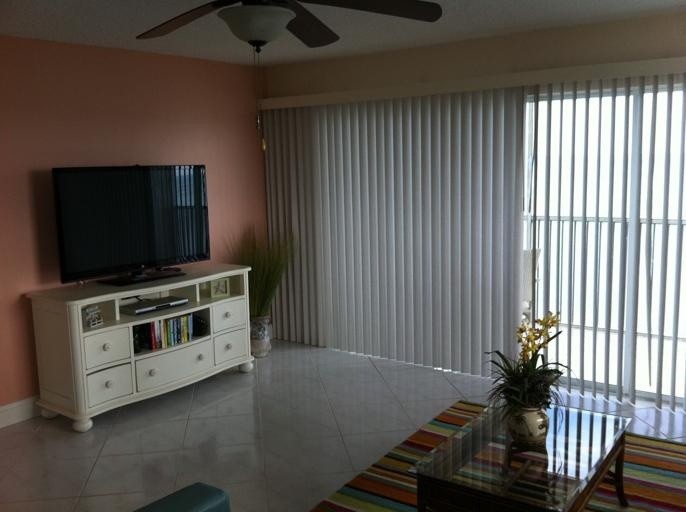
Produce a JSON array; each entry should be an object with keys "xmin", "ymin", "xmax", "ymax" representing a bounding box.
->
[{"xmin": 50, "ymin": 163, "xmax": 211, "ymax": 287}]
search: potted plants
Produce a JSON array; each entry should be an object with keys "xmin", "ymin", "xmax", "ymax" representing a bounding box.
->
[{"xmin": 219, "ymin": 222, "xmax": 301, "ymax": 360}]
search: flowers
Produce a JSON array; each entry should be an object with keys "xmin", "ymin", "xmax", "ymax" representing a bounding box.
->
[{"xmin": 482, "ymin": 302, "xmax": 574, "ymax": 426}]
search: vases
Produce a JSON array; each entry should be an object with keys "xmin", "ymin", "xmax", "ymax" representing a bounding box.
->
[{"xmin": 508, "ymin": 405, "xmax": 550, "ymax": 451}]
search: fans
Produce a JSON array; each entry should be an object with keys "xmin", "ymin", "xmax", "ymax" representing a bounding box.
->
[{"xmin": 136, "ymin": 0, "xmax": 442, "ymax": 152}]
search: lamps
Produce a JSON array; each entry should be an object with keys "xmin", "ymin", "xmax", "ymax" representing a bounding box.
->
[{"xmin": 218, "ymin": 4, "xmax": 298, "ymax": 53}]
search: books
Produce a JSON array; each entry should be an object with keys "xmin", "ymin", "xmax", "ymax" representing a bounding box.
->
[{"xmin": 137, "ymin": 312, "xmax": 193, "ymax": 353}]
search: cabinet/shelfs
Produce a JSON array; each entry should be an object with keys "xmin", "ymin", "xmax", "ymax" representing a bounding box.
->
[{"xmin": 25, "ymin": 261, "xmax": 253, "ymax": 433}]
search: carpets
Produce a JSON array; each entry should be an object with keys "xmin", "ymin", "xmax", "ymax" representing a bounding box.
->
[{"xmin": 311, "ymin": 399, "xmax": 686, "ymax": 512}]
